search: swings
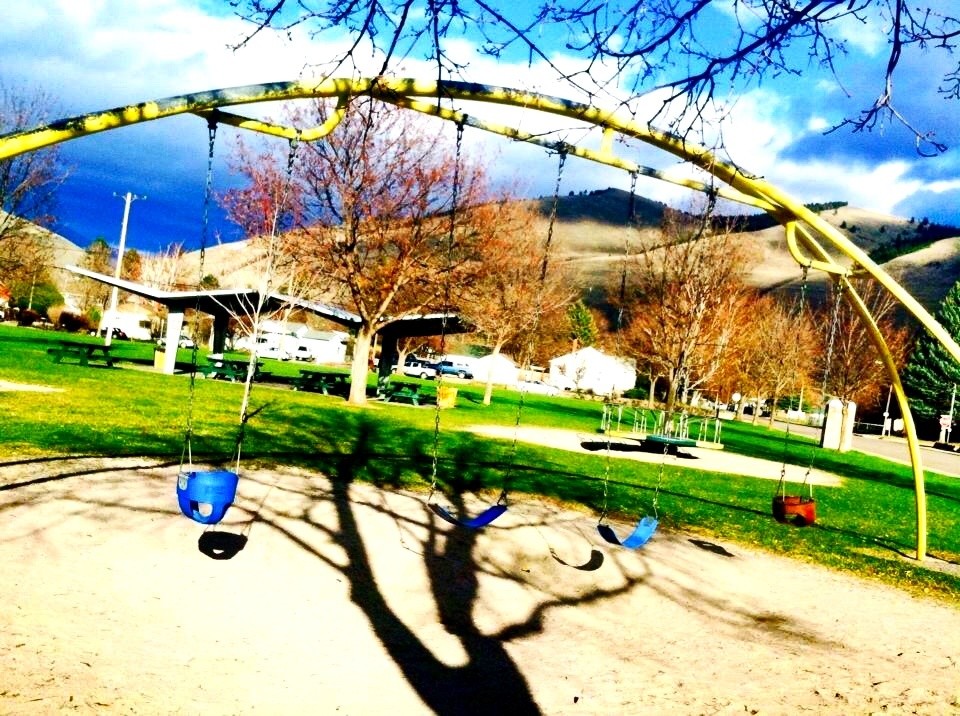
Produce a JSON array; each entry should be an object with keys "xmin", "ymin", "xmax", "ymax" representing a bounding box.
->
[
  {"xmin": 596, "ymin": 179, "xmax": 717, "ymax": 550},
  {"xmin": 426, "ymin": 129, "xmax": 566, "ymax": 529},
  {"xmin": 771, "ymin": 270, "xmax": 842, "ymax": 528},
  {"xmin": 177, "ymin": 128, "xmax": 295, "ymax": 526}
]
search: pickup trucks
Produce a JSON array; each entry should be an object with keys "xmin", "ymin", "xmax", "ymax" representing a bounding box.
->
[
  {"xmin": 391, "ymin": 361, "xmax": 437, "ymax": 380},
  {"xmin": 426, "ymin": 361, "xmax": 474, "ymax": 379}
]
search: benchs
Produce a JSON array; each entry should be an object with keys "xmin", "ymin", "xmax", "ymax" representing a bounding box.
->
[
  {"xmin": 211, "ymin": 368, "xmax": 245, "ymax": 376},
  {"xmin": 380, "ymin": 390, "xmax": 422, "ymax": 400},
  {"xmin": 48, "ymin": 348, "xmax": 121, "ymax": 363},
  {"xmin": 294, "ymin": 379, "xmax": 335, "ymax": 388}
]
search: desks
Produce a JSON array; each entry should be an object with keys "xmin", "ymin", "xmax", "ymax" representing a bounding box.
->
[
  {"xmin": 291, "ymin": 370, "xmax": 350, "ymax": 395},
  {"xmin": 379, "ymin": 382, "xmax": 421, "ymax": 406},
  {"xmin": 648, "ymin": 435, "xmax": 697, "ymax": 447},
  {"xmin": 208, "ymin": 358, "xmax": 262, "ymax": 382},
  {"xmin": 49, "ymin": 341, "xmax": 115, "ymax": 368}
]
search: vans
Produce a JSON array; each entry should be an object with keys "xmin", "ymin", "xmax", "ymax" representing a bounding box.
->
[{"xmin": 101, "ymin": 311, "xmax": 156, "ymax": 340}]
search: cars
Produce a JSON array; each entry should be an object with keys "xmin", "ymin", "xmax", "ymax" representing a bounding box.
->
[
  {"xmin": 251, "ymin": 337, "xmax": 319, "ymax": 362},
  {"xmin": 89, "ymin": 327, "xmax": 129, "ymax": 340},
  {"xmin": 156, "ymin": 335, "xmax": 196, "ymax": 348}
]
